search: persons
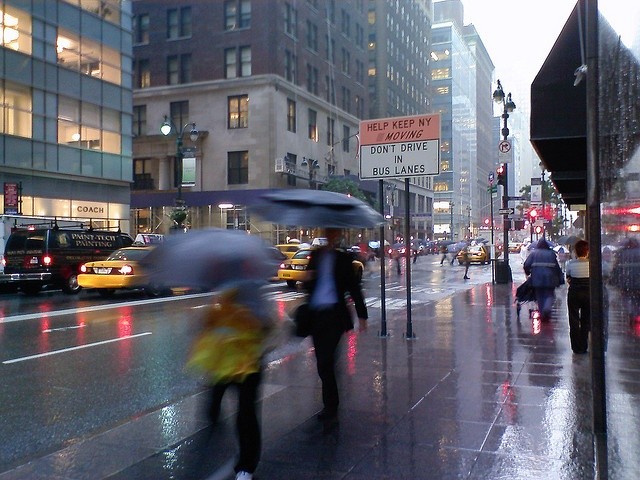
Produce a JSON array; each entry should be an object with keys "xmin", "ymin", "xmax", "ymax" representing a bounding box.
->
[
  {"xmin": 440, "ymin": 244, "xmax": 450, "ymax": 266},
  {"xmin": 462, "ymin": 243, "xmax": 472, "ymax": 280},
  {"xmin": 568, "ymin": 244, "xmax": 576, "ymax": 260},
  {"xmin": 390, "ymin": 250, "xmax": 401, "ymax": 275},
  {"xmin": 566, "ymin": 239, "xmax": 590, "ymax": 354},
  {"xmin": 600, "ymin": 237, "xmax": 640, "ymax": 354},
  {"xmin": 497, "ymin": 238, "xmax": 503, "ymax": 245},
  {"xmin": 522, "ymin": 237, "xmax": 563, "ymax": 320},
  {"xmin": 558, "ymin": 246, "xmax": 564, "ymax": 272},
  {"xmin": 410, "ymin": 236, "xmax": 435, "ymax": 264},
  {"xmin": 198, "ymin": 289, "xmax": 292, "ymax": 480},
  {"xmin": 297, "ymin": 226, "xmax": 368, "ymax": 430}
]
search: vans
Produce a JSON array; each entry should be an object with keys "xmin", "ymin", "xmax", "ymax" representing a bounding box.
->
[{"xmin": 0, "ymin": 216, "xmax": 135, "ymax": 294}]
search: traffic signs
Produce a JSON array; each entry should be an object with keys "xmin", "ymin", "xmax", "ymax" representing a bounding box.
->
[{"xmin": 498, "ymin": 208, "xmax": 514, "ymax": 214}]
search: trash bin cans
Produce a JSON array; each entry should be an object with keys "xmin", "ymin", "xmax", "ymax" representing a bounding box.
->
[{"xmin": 495, "ymin": 258, "xmax": 510, "ymax": 284}]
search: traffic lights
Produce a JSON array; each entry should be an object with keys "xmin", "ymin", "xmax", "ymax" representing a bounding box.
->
[
  {"xmin": 497, "ymin": 166, "xmax": 504, "ymax": 185},
  {"xmin": 484, "ymin": 218, "xmax": 491, "ymax": 226},
  {"xmin": 539, "ymin": 162, "xmax": 546, "ymax": 238},
  {"xmin": 529, "ymin": 208, "xmax": 539, "ymax": 218},
  {"xmin": 534, "ymin": 226, "xmax": 543, "ymax": 234}
]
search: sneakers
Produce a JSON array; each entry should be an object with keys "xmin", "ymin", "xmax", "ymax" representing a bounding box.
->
[{"xmin": 235, "ymin": 469, "xmax": 253, "ymax": 480}]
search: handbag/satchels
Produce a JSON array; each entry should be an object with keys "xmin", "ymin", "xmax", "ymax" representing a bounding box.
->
[{"xmin": 291, "ymin": 302, "xmax": 312, "ymax": 340}]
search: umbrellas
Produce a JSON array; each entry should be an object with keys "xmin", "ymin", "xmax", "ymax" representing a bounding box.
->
[
  {"xmin": 239, "ymin": 187, "xmax": 391, "ymax": 230},
  {"xmin": 390, "ymin": 243, "xmax": 404, "ymax": 250},
  {"xmin": 368, "ymin": 240, "xmax": 379, "ymax": 249},
  {"xmin": 527, "ymin": 239, "xmax": 556, "ymax": 250},
  {"xmin": 134, "ymin": 229, "xmax": 290, "ymax": 296},
  {"xmin": 436, "ymin": 240, "xmax": 455, "ymax": 246},
  {"xmin": 447, "ymin": 243, "xmax": 465, "ymax": 252}
]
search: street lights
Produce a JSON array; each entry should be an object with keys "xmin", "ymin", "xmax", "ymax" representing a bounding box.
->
[
  {"xmin": 493, "ymin": 79, "xmax": 516, "ymax": 282},
  {"xmin": 387, "ymin": 184, "xmax": 397, "ymax": 242},
  {"xmin": 449, "ymin": 201, "xmax": 455, "ymax": 239},
  {"xmin": 301, "ymin": 157, "xmax": 320, "ymax": 189},
  {"xmin": 160, "ymin": 115, "xmax": 199, "ymax": 234}
]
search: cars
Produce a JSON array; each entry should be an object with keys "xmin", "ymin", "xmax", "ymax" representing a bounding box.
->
[
  {"xmin": 388, "ymin": 238, "xmax": 455, "ymax": 258},
  {"xmin": 457, "ymin": 241, "xmax": 489, "ymax": 264},
  {"xmin": 276, "ymin": 239, "xmax": 313, "ymax": 258},
  {"xmin": 77, "ymin": 234, "xmax": 192, "ymax": 297},
  {"xmin": 508, "ymin": 243, "xmax": 521, "ymax": 253},
  {"xmin": 278, "ymin": 238, "xmax": 364, "ymax": 288}
]
